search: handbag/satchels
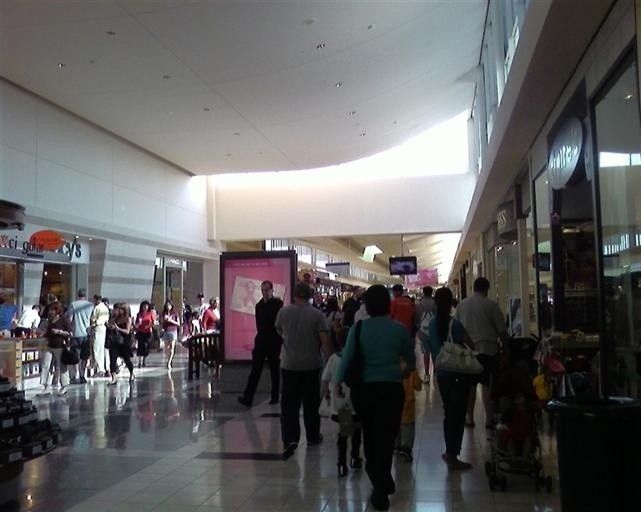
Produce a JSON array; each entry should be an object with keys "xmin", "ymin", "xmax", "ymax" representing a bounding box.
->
[
  {"xmin": 60, "ymin": 347, "xmax": 79, "ymax": 365},
  {"xmin": 105, "ymin": 332, "xmax": 124, "ymax": 349},
  {"xmin": 435, "ymin": 340, "xmax": 485, "ymax": 374}
]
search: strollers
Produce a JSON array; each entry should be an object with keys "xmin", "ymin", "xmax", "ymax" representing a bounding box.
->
[{"xmin": 479, "ymin": 332, "xmax": 557, "ymax": 493}]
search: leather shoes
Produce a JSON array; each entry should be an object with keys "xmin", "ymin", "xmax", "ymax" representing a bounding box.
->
[
  {"xmin": 107, "ymin": 381, "xmax": 117, "ymax": 385},
  {"xmin": 129, "ymin": 375, "xmax": 135, "ymax": 382}
]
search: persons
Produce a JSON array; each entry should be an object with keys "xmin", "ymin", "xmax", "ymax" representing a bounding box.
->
[
  {"xmin": 238, "ymin": 279, "xmax": 510, "ymax": 512},
  {"xmin": 9, "ymin": 288, "xmax": 222, "ymax": 397}
]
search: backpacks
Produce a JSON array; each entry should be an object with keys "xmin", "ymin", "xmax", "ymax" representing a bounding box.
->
[{"xmin": 420, "ymin": 312, "xmax": 435, "ymax": 335}]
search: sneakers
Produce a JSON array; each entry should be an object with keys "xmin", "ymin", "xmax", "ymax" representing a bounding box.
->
[
  {"xmin": 36, "ymin": 390, "xmax": 51, "ymax": 396},
  {"xmin": 237, "ymin": 397, "xmax": 252, "ymax": 408},
  {"xmin": 56, "ymin": 385, "xmax": 72, "ymax": 396},
  {"xmin": 138, "ymin": 363, "xmax": 145, "ymax": 368},
  {"xmin": 466, "ymin": 414, "xmax": 475, "ymax": 427},
  {"xmin": 269, "ymin": 399, "xmax": 280, "ymax": 404},
  {"xmin": 281, "ymin": 442, "xmax": 298, "ymax": 459},
  {"xmin": 307, "ymin": 433, "xmax": 323, "ymax": 445},
  {"xmin": 167, "ymin": 360, "xmax": 172, "ymax": 369},
  {"xmin": 393, "ymin": 446, "xmax": 413, "ymax": 462},
  {"xmin": 338, "ymin": 458, "xmax": 364, "ymax": 477},
  {"xmin": 69, "ymin": 371, "xmax": 112, "ymax": 384},
  {"xmin": 442, "ymin": 453, "xmax": 472, "ymax": 470}
]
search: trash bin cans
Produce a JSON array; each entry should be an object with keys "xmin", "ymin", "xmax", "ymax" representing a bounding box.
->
[{"xmin": 546, "ymin": 394, "xmax": 641, "ymax": 512}]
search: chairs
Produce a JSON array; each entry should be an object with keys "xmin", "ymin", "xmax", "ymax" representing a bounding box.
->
[{"xmin": 188, "ymin": 333, "xmax": 221, "ymax": 380}]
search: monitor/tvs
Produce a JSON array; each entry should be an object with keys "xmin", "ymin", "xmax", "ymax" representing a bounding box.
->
[
  {"xmin": 536, "ymin": 252, "xmax": 550, "ymax": 271},
  {"xmin": 389, "ymin": 256, "xmax": 417, "ymax": 275}
]
